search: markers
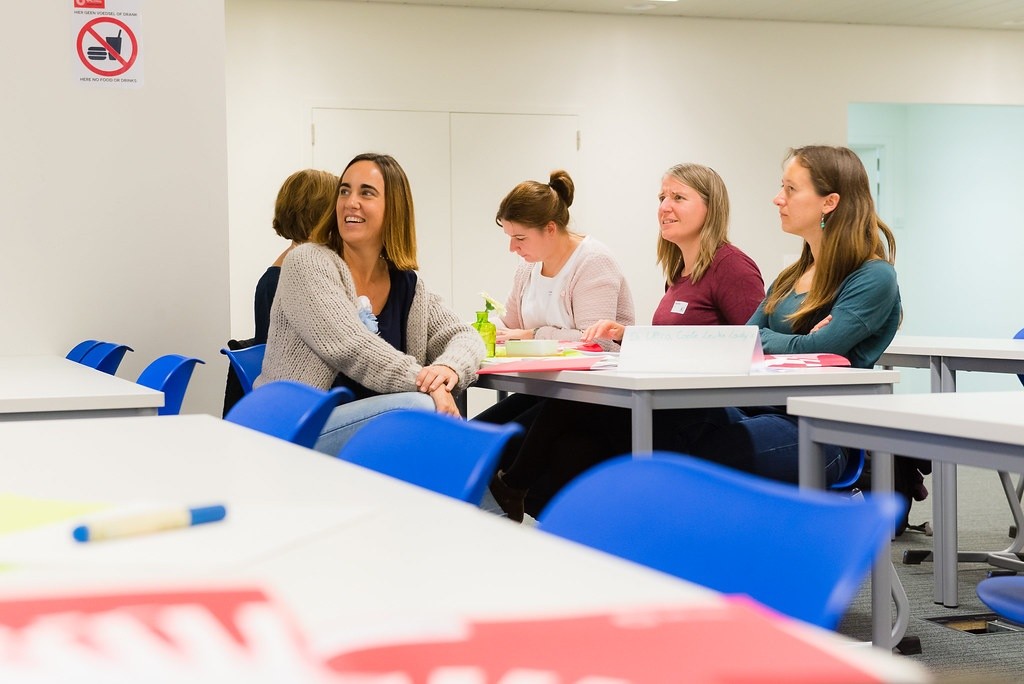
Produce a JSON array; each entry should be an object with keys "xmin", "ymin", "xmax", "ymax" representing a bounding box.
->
[{"xmin": 71, "ymin": 503, "xmax": 227, "ymax": 543}]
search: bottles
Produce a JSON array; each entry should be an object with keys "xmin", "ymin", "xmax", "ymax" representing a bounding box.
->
[{"xmin": 471, "ymin": 311, "xmax": 496, "ymax": 358}]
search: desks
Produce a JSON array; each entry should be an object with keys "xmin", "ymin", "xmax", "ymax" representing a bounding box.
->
[
  {"xmin": 0, "ymin": 414, "xmax": 930, "ymax": 684},
  {"xmin": 784, "ymin": 389, "xmax": 1024, "ymax": 658},
  {"xmin": 449, "ymin": 338, "xmax": 900, "ymax": 662},
  {"xmin": 1, "ymin": 355, "xmax": 166, "ymax": 420},
  {"xmin": 872, "ymin": 333, "xmax": 1024, "ymax": 551}
]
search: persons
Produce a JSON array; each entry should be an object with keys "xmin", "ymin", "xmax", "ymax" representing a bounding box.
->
[
  {"xmin": 254, "ymin": 153, "xmax": 509, "ymax": 518},
  {"xmin": 467, "ymin": 147, "xmax": 903, "ymax": 524}
]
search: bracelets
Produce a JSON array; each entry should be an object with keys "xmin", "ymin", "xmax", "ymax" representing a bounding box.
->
[{"xmin": 532, "ymin": 327, "xmax": 540, "ymax": 339}]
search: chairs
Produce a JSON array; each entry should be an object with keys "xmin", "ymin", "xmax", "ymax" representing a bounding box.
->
[{"xmin": 64, "ymin": 339, "xmax": 1024, "ymax": 638}]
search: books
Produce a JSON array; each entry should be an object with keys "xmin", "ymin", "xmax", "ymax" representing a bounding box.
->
[
  {"xmin": 1, "ymin": 584, "xmax": 886, "ymax": 684},
  {"xmin": 476, "ymin": 356, "xmax": 611, "ymax": 375}
]
